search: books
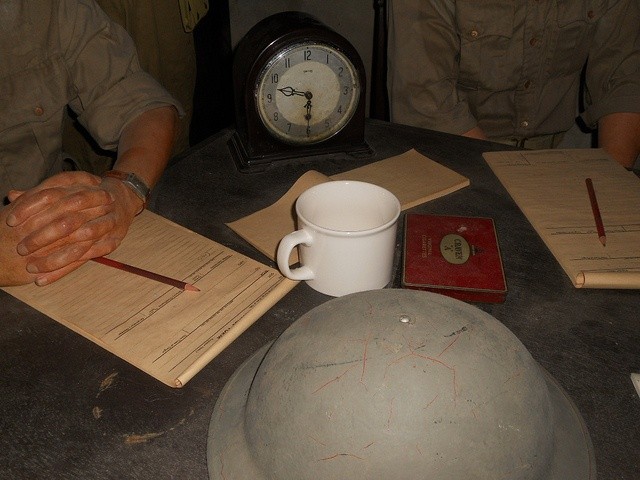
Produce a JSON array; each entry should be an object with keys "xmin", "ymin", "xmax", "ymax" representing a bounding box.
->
[
  {"xmin": 0, "ymin": 207, "xmax": 303, "ymax": 390},
  {"xmin": 225, "ymin": 148, "xmax": 470, "ymax": 269},
  {"xmin": 482, "ymin": 147, "xmax": 640, "ymax": 290}
]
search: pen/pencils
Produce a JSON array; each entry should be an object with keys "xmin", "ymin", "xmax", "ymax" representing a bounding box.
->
[
  {"xmin": 89, "ymin": 256, "xmax": 199, "ymax": 290},
  {"xmin": 584, "ymin": 179, "xmax": 604, "ymax": 248}
]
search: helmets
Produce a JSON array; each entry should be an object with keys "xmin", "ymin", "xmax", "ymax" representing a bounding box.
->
[{"xmin": 205, "ymin": 288, "xmax": 598, "ymax": 480}]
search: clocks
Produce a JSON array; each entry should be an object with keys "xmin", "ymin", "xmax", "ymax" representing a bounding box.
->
[{"xmin": 252, "ymin": 37, "xmax": 363, "ymax": 145}]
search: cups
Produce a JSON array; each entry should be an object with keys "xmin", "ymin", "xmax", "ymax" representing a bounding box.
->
[{"xmin": 276, "ymin": 181, "xmax": 401, "ymax": 298}]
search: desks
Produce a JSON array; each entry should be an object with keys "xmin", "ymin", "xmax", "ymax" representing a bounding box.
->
[{"xmin": 3, "ymin": 116, "xmax": 639, "ymax": 479}]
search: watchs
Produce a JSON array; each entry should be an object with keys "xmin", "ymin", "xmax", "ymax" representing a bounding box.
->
[{"xmin": 105, "ymin": 169, "xmax": 152, "ymax": 217}]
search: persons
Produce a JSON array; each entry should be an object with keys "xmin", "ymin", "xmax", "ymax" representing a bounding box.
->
[
  {"xmin": 0, "ymin": 1, "xmax": 188, "ymax": 286},
  {"xmin": 388, "ymin": 1, "xmax": 640, "ymax": 168}
]
